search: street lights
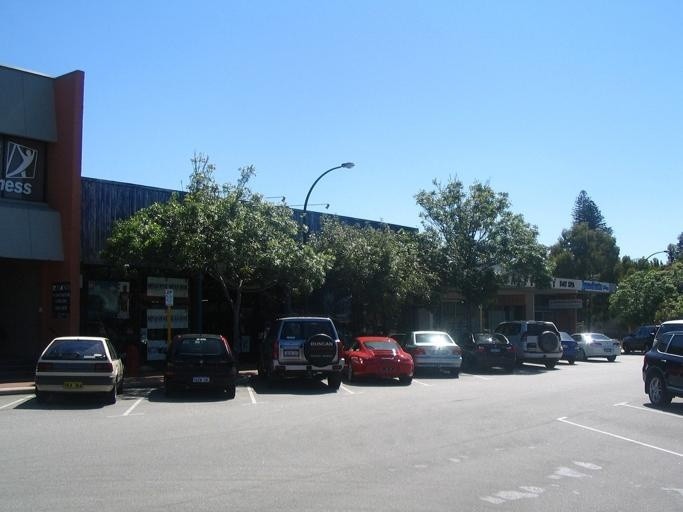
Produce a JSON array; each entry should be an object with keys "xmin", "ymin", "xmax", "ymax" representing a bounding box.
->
[
  {"xmin": 637, "ymin": 247, "xmax": 668, "ymax": 271},
  {"xmin": 296, "ymin": 159, "xmax": 354, "ymax": 316}
]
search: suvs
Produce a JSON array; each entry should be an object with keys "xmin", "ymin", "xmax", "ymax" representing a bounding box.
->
[{"xmin": 251, "ymin": 314, "xmax": 343, "ymax": 394}]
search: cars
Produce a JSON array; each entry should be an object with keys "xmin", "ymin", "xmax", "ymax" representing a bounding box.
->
[
  {"xmin": 162, "ymin": 328, "xmax": 239, "ymax": 401},
  {"xmin": 32, "ymin": 333, "xmax": 127, "ymax": 410}
]
